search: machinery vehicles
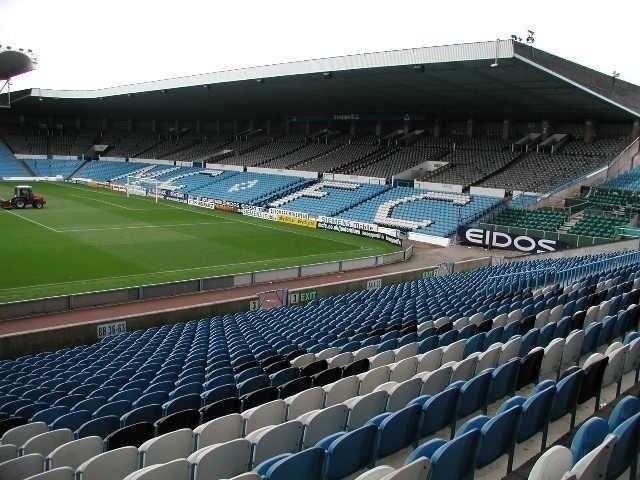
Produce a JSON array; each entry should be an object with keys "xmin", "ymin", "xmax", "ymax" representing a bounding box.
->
[{"xmin": 0, "ymin": 185, "xmax": 47, "ymax": 210}]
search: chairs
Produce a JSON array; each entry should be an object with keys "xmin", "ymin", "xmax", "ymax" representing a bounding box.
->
[
  {"xmin": 486, "ymin": 164, "xmax": 640, "ymax": 239},
  {"xmin": 0, "ymin": 251, "xmax": 640, "ymax": 479}
]
